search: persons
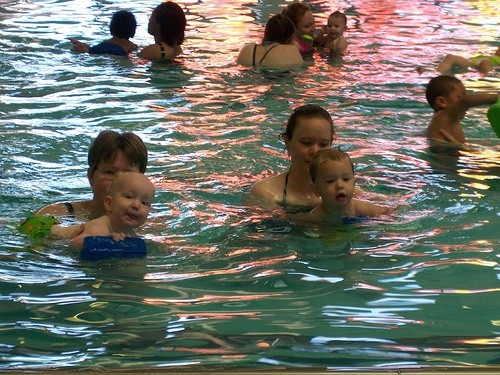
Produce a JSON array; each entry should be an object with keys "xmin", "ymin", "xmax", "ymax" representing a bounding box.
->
[
  {"xmin": 137, "ymin": 1, "xmax": 186, "ymax": 59},
  {"xmin": 70, "ymin": 10, "xmax": 138, "ymax": 55},
  {"xmin": 250, "ymin": 104, "xmax": 410, "ymax": 205},
  {"xmin": 23, "ymin": 130, "xmax": 148, "ymax": 237},
  {"xmin": 425, "ymin": 75, "xmax": 500, "ymax": 143},
  {"xmin": 237, "ymin": 14, "xmax": 304, "ymax": 66},
  {"xmin": 305, "ymin": 148, "xmax": 396, "ymax": 218},
  {"xmin": 69, "ymin": 171, "xmax": 155, "ymax": 250},
  {"xmin": 281, "ymin": 3, "xmax": 317, "ymax": 57},
  {"xmin": 435, "ymin": 47, "xmax": 500, "ymax": 72},
  {"xmin": 317, "ymin": 11, "xmax": 348, "ymax": 54}
]
